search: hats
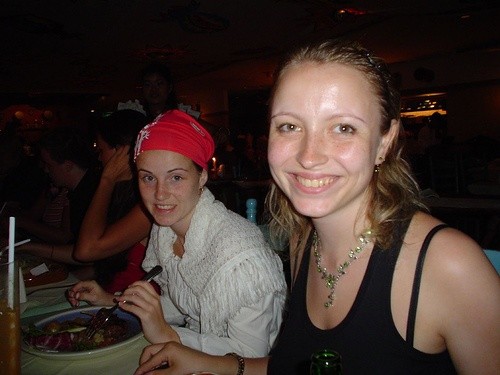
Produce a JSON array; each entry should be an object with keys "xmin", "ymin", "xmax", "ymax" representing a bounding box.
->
[{"xmin": 133, "ymin": 109, "xmax": 214, "ymax": 171}]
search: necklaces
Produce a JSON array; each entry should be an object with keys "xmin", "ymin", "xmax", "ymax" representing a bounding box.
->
[{"xmin": 311, "ymin": 227, "xmax": 372, "ymax": 309}]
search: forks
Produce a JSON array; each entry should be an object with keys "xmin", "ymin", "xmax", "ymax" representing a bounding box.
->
[{"xmin": 85, "ymin": 264, "xmax": 162, "ymax": 341}]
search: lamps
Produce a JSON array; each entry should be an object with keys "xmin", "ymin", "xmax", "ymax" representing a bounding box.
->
[{"xmin": 400, "ymin": 98, "xmax": 448, "ymax": 117}]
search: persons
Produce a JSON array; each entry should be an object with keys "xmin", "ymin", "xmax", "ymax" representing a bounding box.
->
[
  {"xmin": 196, "ymin": 103, "xmax": 499, "ymax": 204},
  {"xmin": 132, "ymin": 41, "xmax": 499, "ymax": 375},
  {"xmin": 1, "ymin": 125, "xmax": 128, "ymax": 285},
  {"xmin": 65, "ymin": 104, "xmax": 287, "ymax": 357},
  {"xmin": 0, "ymin": 110, "xmax": 162, "ymax": 299}
]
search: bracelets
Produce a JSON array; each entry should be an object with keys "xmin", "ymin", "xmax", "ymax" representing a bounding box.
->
[
  {"xmin": 50, "ymin": 244, "xmax": 54, "ymax": 260},
  {"xmin": 222, "ymin": 352, "xmax": 246, "ymax": 375},
  {"xmin": 46, "ymin": 244, "xmax": 51, "ymax": 259}
]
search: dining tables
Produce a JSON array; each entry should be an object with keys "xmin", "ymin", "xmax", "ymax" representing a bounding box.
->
[{"xmin": 0, "ymin": 256, "xmax": 152, "ymax": 375}]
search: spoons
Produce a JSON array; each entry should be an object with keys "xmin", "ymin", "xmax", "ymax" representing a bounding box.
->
[{"xmin": 0, "ymin": 239, "xmax": 31, "ymax": 258}]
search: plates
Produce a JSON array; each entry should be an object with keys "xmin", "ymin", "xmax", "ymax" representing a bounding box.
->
[
  {"xmin": 20, "ymin": 307, "xmax": 145, "ymax": 359},
  {"xmin": 15, "ymin": 267, "xmax": 79, "ymax": 290}
]
